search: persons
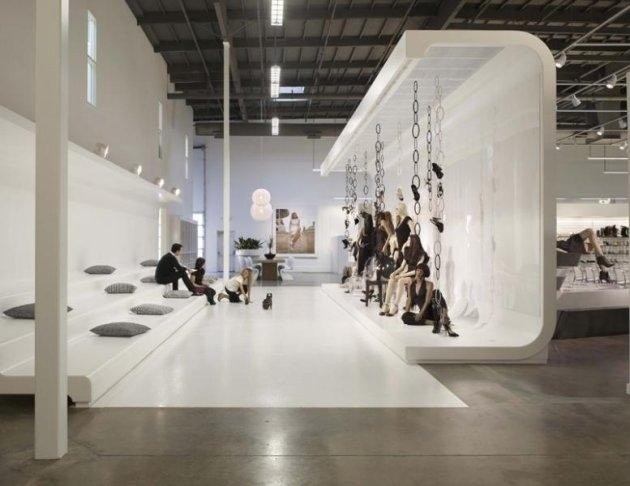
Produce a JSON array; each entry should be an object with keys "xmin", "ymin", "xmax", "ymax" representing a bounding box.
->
[
  {"xmin": 406, "ymin": 264, "xmax": 460, "ymax": 336},
  {"xmin": 353, "ymin": 202, "xmax": 429, "ymax": 316},
  {"xmin": 558, "ymin": 227, "xmax": 617, "ymax": 286},
  {"xmin": 218, "ymin": 268, "xmax": 253, "ymax": 302},
  {"xmin": 155, "ymin": 243, "xmax": 204, "ymax": 294},
  {"xmin": 191, "ymin": 257, "xmax": 216, "ymax": 304},
  {"xmin": 287, "ymin": 212, "xmax": 303, "ymax": 252}
]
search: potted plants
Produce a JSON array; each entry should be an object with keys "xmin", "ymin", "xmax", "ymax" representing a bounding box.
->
[{"xmin": 231, "ymin": 237, "xmax": 266, "ymax": 257}]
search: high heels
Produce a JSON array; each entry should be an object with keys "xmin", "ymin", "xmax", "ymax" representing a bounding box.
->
[
  {"xmin": 263, "ymin": 293, "xmax": 272, "ymax": 310},
  {"xmin": 599, "ymin": 271, "xmax": 613, "ymax": 283},
  {"xmin": 360, "ymin": 290, "xmax": 374, "ymax": 302},
  {"xmin": 379, "ymin": 309, "xmax": 398, "ymax": 317},
  {"xmin": 444, "ymin": 324, "xmax": 458, "ymax": 337},
  {"xmin": 596, "ymin": 256, "xmax": 615, "ymax": 269}
]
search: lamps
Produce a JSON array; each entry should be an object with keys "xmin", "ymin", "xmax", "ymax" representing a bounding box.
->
[
  {"xmin": 572, "ymin": 93, "xmax": 581, "ymax": 108},
  {"xmin": 606, "ymin": 74, "xmax": 618, "ymax": 89},
  {"xmin": 554, "ymin": 52, "xmax": 566, "ymax": 69}
]
